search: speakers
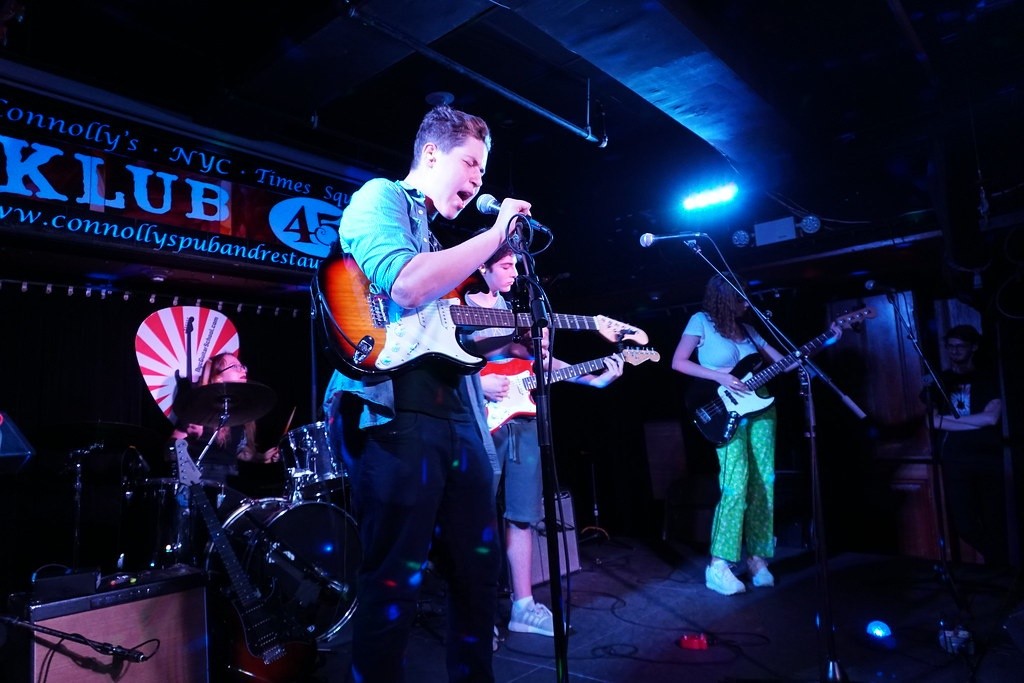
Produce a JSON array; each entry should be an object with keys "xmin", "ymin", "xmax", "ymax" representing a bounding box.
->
[
  {"xmin": 0, "ymin": 564, "xmax": 211, "ymax": 682},
  {"xmin": 503, "ymin": 492, "xmax": 582, "ymax": 595}
]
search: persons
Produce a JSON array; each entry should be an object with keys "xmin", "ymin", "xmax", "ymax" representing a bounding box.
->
[
  {"xmin": 671, "ymin": 271, "xmax": 842, "ymax": 595},
  {"xmin": 463, "ymin": 244, "xmax": 625, "ymax": 650},
  {"xmin": 172, "ymin": 352, "xmax": 279, "ymax": 485},
  {"xmin": 926, "ymin": 325, "xmax": 1009, "ymax": 595},
  {"xmin": 339, "ymin": 107, "xmax": 549, "ymax": 683}
]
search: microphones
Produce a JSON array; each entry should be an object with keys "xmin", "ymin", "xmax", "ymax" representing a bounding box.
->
[
  {"xmin": 865, "ymin": 280, "xmax": 903, "ymax": 293},
  {"xmin": 119, "ymin": 647, "xmax": 147, "ymax": 663},
  {"xmin": 640, "ymin": 233, "xmax": 707, "ymax": 248},
  {"xmin": 476, "ymin": 194, "xmax": 550, "ymax": 234},
  {"xmin": 331, "ymin": 581, "xmax": 351, "ymax": 596},
  {"xmin": 557, "ymin": 523, "xmax": 574, "ymax": 533}
]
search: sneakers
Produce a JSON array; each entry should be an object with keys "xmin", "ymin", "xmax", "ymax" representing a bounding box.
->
[
  {"xmin": 746, "ymin": 556, "xmax": 774, "ymax": 587},
  {"xmin": 507, "ymin": 593, "xmax": 566, "ymax": 636},
  {"xmin": 705, "ymin": 560, "xmax": 746, "ymax": 595}
]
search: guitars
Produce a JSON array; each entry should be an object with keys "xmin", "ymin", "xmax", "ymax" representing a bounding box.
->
[
  {"xmin": 173, "ymin": 437, "xmax": 288, "ymax": 666},
  {"xmin": 314, "ymin": 253, "xmax": 650, "ymax": 385},
  {"xmin": 481, "ymin": 344, "xmax": 664, "ymax": 438},
  {"xmin": 682, "ymin": 300, "xmax": 878, "ymax": 449}
]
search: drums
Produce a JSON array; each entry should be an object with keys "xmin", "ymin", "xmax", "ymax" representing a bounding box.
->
[{"xmin": 174, "ymin": 412, "xmax": 356, "ymax": 654}]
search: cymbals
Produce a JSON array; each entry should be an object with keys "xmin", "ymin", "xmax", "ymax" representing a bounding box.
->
[{"xmin": 170, "ymin": 380, "xmax": 280, "ymax": 428}]
